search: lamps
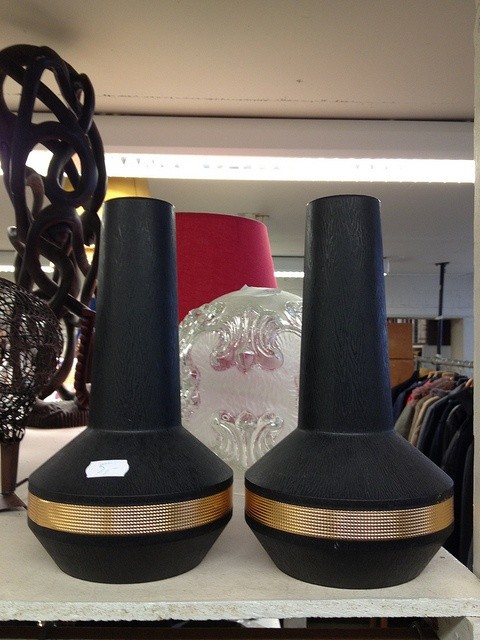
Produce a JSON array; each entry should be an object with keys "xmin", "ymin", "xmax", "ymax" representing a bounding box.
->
[
  {"xmin": 383, "ymin": 257, "xmax": 391, "ymax": 277},
  {"xmin": 63, "ymin": 179, "xmax": 151, "ymax": 254},
  {"xmin": 0, "ymin": 277, "xmax": 64, "ymax": 513}
]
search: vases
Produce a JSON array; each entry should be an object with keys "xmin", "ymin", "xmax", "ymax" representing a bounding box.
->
[
  {"xmin": 27, "ymin": 198, "xmax": 234, "ymax": 585},
  {"xmin": 243, "ymin": 194, "xmax": 455, "ymax": 590}
]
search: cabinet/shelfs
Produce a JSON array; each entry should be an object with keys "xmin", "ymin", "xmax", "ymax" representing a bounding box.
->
[{"xmin": 387, "ymin": 323, "xmax": 413, "ymax": 390}]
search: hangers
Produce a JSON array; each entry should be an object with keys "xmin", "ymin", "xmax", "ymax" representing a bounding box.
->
[{"xmin": 408, "ymin": 352, "xmax": 475, "ymax": 399}]
